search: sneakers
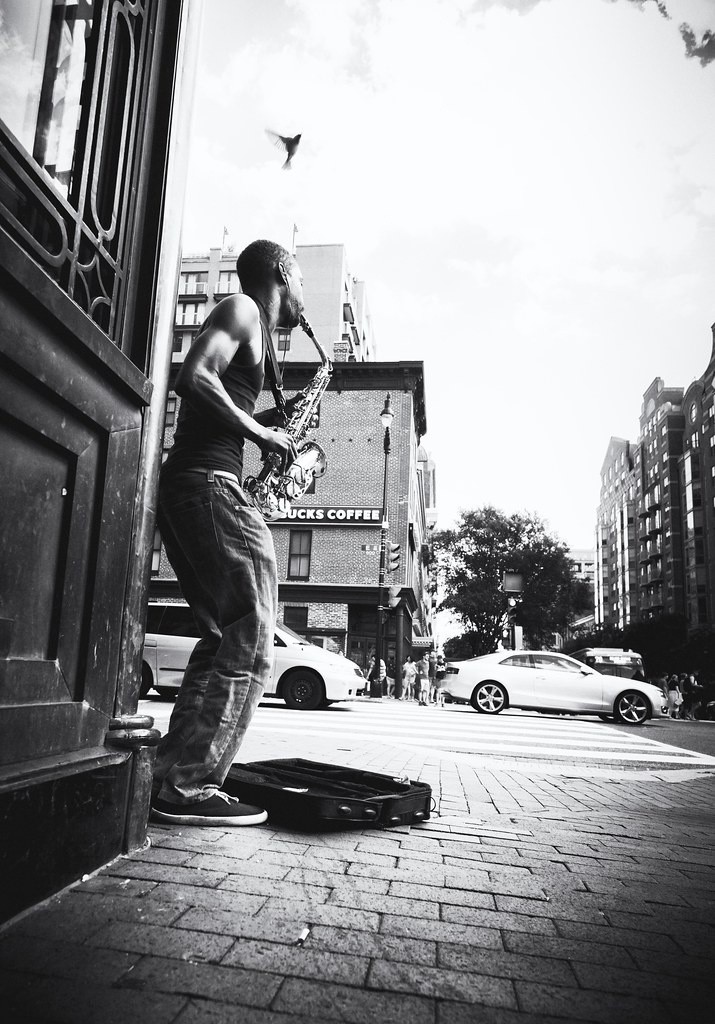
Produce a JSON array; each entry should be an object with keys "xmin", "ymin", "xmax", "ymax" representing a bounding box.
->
[{"xmin": 149, "ymin": 789, "xmax": 268, "ymax": 826}]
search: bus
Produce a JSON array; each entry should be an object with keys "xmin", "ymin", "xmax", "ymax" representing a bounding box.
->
[{"xmin": 567, "ymin": 647, "xmax": 646, "ymax": 680}]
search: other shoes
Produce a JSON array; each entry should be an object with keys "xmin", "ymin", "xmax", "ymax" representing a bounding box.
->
[{"xmin": 418, "ymin": 701, "xmax": 429, "ymax": 706}]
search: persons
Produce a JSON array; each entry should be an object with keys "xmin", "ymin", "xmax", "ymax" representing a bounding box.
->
[
  {"xmin": 366, "ymin": 655, "xmax": 396, "ymax": 699},
  {"xmin": 658, "ymin": 671, "xmax": 704, "ymax": 721},
  {"xmin": 398, "ymin": 651, "xmax": 447, "ymax": 707},
  {"xmin": 150, "ymin": 240, "xmax": 320, "ymax": 829}
]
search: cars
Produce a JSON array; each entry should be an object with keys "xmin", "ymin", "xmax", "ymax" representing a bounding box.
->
[
  {"xmin": 140, "ymin": 602, "xmax": 372, "ymax": 709},
  {"xmin": 438, "ymin": 648, "xmax": 674, "ymax": 725}
]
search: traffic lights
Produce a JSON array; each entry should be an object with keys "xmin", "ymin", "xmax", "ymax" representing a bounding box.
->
[
  {"xmin": 387, "ymin": 586, "xmax": 402, "ymax": 608},
  {"xmin": 387, "ymin": 541, "xmax": 400, "ymax": 571},
  {"xmin": 508, "ymin": 597, "xmax": 517, "ymax": 626},
  {"xmin": 502, "ymin": 570, "xmax": 524, "ymax": 592}
]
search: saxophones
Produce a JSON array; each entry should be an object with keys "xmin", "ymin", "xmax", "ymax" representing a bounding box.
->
[{"xmin": 241, "ymin": 312, "xmax": 337, "ymax": 523}]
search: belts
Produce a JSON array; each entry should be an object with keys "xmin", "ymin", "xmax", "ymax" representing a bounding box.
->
[{"xmin": 187, "ymin": 466, "xmax": 240, "ymax": 484}]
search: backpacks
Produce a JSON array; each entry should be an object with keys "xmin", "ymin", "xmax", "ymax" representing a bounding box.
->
[{"xmin": 683, "ymin": 678, "xmax": 695, "ymax": 691}]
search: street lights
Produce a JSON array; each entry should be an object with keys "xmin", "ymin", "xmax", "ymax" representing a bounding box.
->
[{"xmin": 372, "ymin": 389, "xmax": 395, "ymax": 701}]
